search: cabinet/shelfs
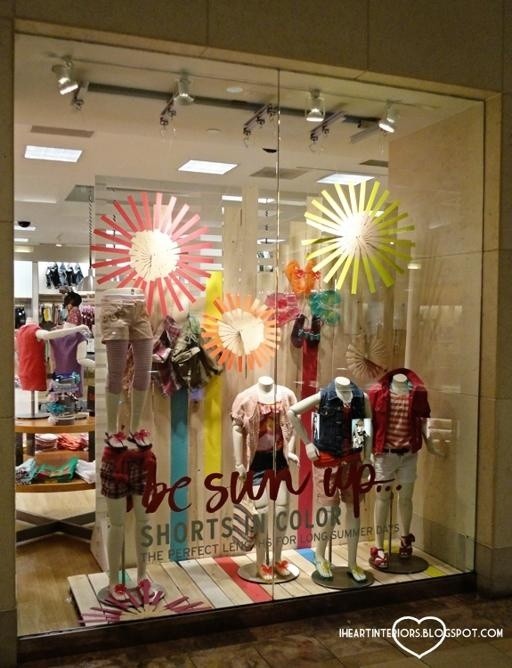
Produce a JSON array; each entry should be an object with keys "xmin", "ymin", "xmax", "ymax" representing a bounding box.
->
[
  {"xmin": 32, "ymin": 243, "xmax": 95, "ymax": 374},
  {"xmin": 14, "ymin": 414, "xmax": 94, "ymax": 544}
]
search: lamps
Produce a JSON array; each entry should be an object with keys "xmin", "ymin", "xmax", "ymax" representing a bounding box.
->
[
  {"xmin": 22, "ymin": 144, "xmax": 83, "ymax": 164},
  {"xmin": 377, "ymin": 101, "xmax": 399, "ymax": 136},
  {"xmin": 315, "ymin": 170, "xmax": 374, "ymax": 188},
  {"xmin": 303, "ymin": 89, "xmax": 326, "ymax": 124},
  {"xmin": 173, "ymin": 79, "xmax": 194, "ymax": 108},
  {"xmin": 176, "ymin": 158, "xmax": 238, "ymax": 176},
  {"xmin": 50, "ymin": 64, "xmax": 78, "ymax": 96}
]
